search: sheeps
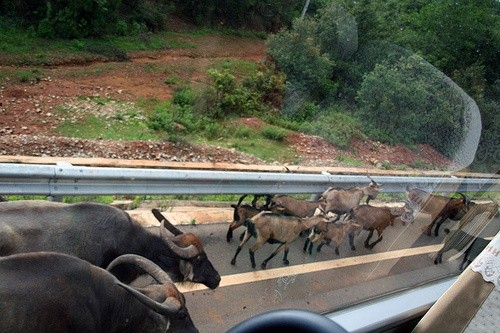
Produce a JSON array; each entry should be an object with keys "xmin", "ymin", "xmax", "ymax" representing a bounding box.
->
[
  {"xmin": 226, "ymin": 174, "xmax": 414, "ymax": 269},
  {"xmin": 434, "ymin": 228, "xmax": 492, "ymax": 271},
  {"xmin": 401, "ymin": 184, "xmax": 498, "ymax": 237}
]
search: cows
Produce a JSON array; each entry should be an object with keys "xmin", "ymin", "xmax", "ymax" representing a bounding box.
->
[{"xmin": 0, "ymin": 200, "xmax": 221, "ymax": 333}]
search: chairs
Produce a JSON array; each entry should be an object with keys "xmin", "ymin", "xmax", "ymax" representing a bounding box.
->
[{"xmin": 215, "ymin": 0, "xmax": 483, "ymax": 296}]
